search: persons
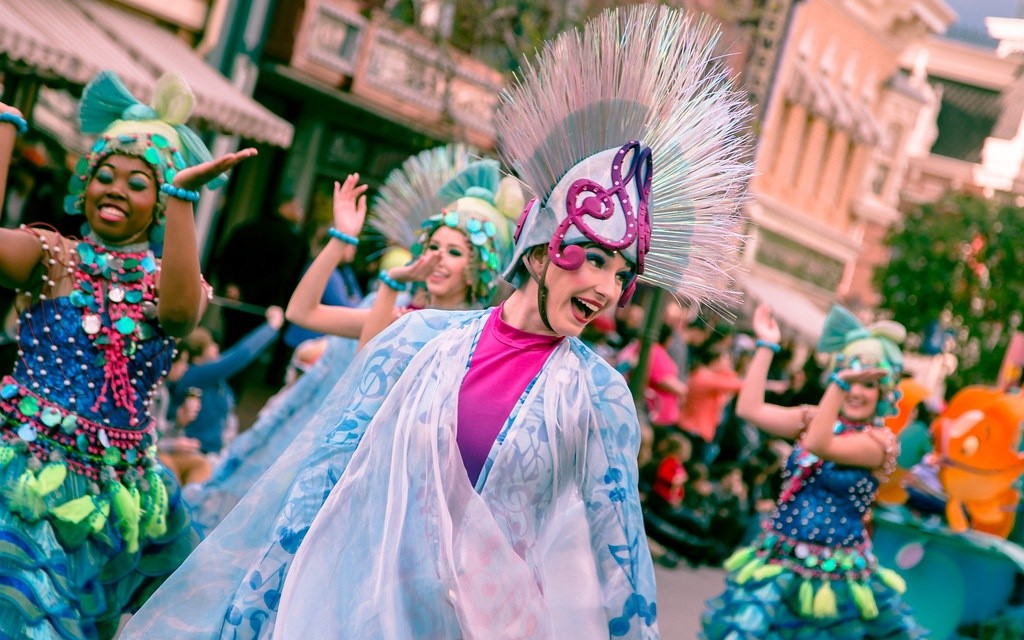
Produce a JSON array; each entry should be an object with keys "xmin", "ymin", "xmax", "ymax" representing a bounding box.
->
[
  {"xmin": 117, "ymin": 140, "xmax": 660, "ymax": 640},
  {"xmin": 696, "ymin": 301, "xmax": 927, "ymax": 640},
  {"xmin": 216, "ymin": 193, "xmax": 311, "ymax": 406},
  {"xmin": 285, "ymin": 171, "xmax": 516, "ymax": 360},
  {"xmin": 575, "ymin": 298, "xmax": 1024, "ymax": 543},
  {"xmin": 0, "ymin": 67, "xmax": 258, "ymax": 640}
]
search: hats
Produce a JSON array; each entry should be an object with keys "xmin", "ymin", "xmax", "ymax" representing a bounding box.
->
[
  {"xmin": 813, "ymin": 303, "xmax": 907, "ymax": 418},
  {"xmin": 64, "ymin": 69, "xmax": 231, "ymax": 246},
  {"xmin": 409, "ymin": 158, "xmax": 524, "ymax": 311}
]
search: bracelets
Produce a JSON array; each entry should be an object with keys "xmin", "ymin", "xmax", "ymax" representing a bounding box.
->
[
  {"xmin": 376, "ymin": 269, "xmax": 407, "ymax": 291},
  {"xmin": 328, "ymin": 227, "xmax": 359, "ymax": 245},
  {"xmin": 755, "ymin": 339, "xmax": 783, "ymax": 356},
  {"xmin": 0, "ymin": 111, "xmax": 28, "ymax": 137},
  {"xmin": 827, "ymin": 372, "xmax": 851, "ymax": 392},
  {"xmin": 160, "ymin": 183, "xmax": 200, "ymax": 203}
]
